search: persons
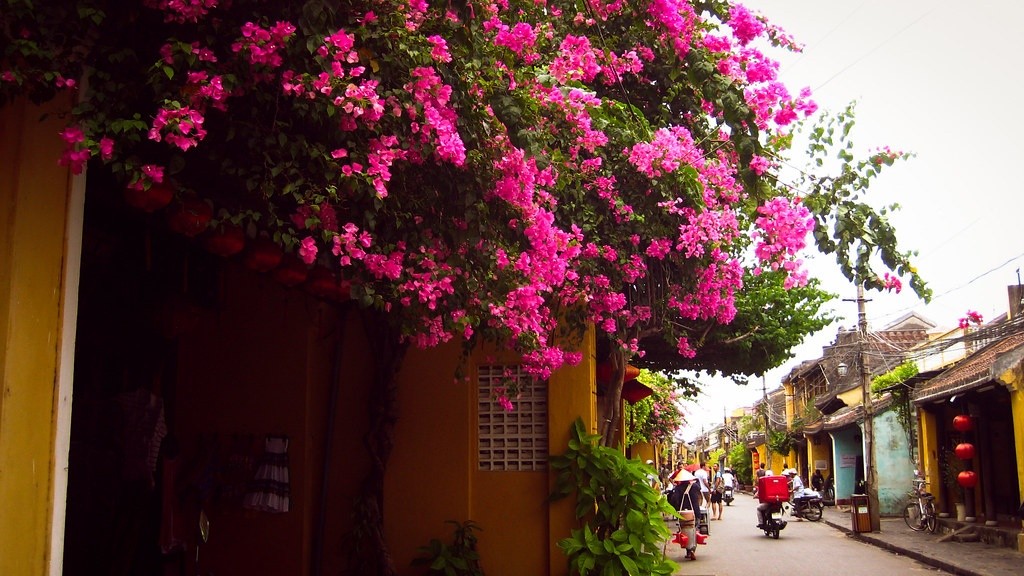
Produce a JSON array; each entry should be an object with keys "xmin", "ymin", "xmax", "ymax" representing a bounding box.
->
[
  {"xmin": 756, "ymin": 464, "xmax": 765, "ymax": 481},
  {"xmin": 643, "ymin": 460, "xmax": 658, "ymax": 488},
  {"xmin": 673, "ymin": 469, "xmax": 700, "ymax": 560},
  {"xmin": 669, "ymin": 462, "xmax": 684, "ymax": 487},
  {"xmin": 812, "ymin": 470, "xmax": 824, "ymax": 490},
  {"xmin": 685, "ymin": 463, "xmax": 692, "ymax": 473},
  {"xmin": 722, "ymin": 464, "xmax": 737, "ymax": 498},
  {"xmin": 660, "ymin": 464, "xmax": 670, "ymax": 491},
  {"xmin": 710, "ymin": 463, "xmax": 723, "ymax": 520},
  {"xmin": 753, "ymin": 470, "xmax": 781, "ymax": 527},
  {"xmin": 781, "ymin": 464, "xmax": 789, "ymax": 475},
  {"xmin": 787, "ymin": 468, "xmax": 804, "ymax": 517},
  {"xmin": 696, "ymin": 462, "xmax": 710, "ymax": 509}
]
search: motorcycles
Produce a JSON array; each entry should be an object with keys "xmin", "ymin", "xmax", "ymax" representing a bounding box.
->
[
  {"xmin": 778, "ymin": 490, "xmax": 824, "ymax": 522},
  {"xmin": 753, "ymin": 487, "xmax": 788, "ymax": 540}
]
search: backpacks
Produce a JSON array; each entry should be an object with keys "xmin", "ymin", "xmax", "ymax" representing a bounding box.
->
[{"xmin": 715, "ymin": 476, "xmax": 725, "ymax": 494}]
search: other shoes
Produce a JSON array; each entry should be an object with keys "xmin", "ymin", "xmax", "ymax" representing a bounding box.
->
[
  {"xmin": 757, "ymin": 524, "xmax": 763, "ymax": 527},
  {"xmin": 797, "ymin": 518, "xmax": 803, "ymax": 522},
  {"xmin": 718, "ymin": 518, "xmax": 722, "ymax": 520},
  {"xmin": 691, "ymin": 551, "xmax": 695, "ymax": 560},
  {"xmin": 685, "ymin": 553, "xmax": 691, "ymax": 558},
  {"xmin": 710, "ymin": 517, "xmax": 716, "ymax": 520}
]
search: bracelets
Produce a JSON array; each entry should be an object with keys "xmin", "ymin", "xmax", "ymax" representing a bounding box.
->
[{"xmin": 714, "ymin": 489, "xmax": 716, "ymax": 490}]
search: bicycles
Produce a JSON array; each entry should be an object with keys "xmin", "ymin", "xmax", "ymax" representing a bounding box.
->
[{"xmin": 904, "ymin": 480, "xmax": 937, "ymax": 533}]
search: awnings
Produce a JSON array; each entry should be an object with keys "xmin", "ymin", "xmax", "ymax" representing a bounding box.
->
[{"xmin": 621, "ymin": 379, "xmax": 653, "ymax": 406}]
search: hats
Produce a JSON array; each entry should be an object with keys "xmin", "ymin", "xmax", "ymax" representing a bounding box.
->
[
  {"xmin": 789, "ymin": 468, "xmax": 797, "ymax": 474},
  {"xmin": 646, "ymin": 460, "xmax": 653, "ymax": 465},
  {"xmin": 674, "ymin": 470, "xmax": 696, "ymax": 481}
]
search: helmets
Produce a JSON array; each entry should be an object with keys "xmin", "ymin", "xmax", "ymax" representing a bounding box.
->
[
  {"xmin": 724, "ymin": 467, "xmax": 730, "ymax": 471},
  {"xmin": 765, "ymin": 470, "xmax": 773, "ymax": 477}
]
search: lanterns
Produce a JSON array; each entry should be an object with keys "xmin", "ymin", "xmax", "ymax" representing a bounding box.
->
[
  {"xmin": 953, "ymin": 415, "xmax": 973, "ymax": 432},
  {"xmin": 598, "ymin": 361, "xmax": 641, "ymax": 385},
  {"xmin": 753, "ymin": 453, "xmax": 760, "ymax": 470},
  {"xmin": 958, "ymin": 471, "xmax": 976, "ymax": 487},
  {"xmin": 955, "ymin": 442, "xmax": 976, "ymax": 460}
]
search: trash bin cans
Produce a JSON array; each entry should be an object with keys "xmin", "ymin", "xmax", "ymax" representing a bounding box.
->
[{"xmin": 851, "ymin": 495, "xmax": 872, "ymax": 532}]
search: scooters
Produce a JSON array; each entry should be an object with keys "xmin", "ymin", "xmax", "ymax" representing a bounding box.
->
[{"xmin": 721, "ymin": 485, "xmax": 735, "ymax": 506}]
